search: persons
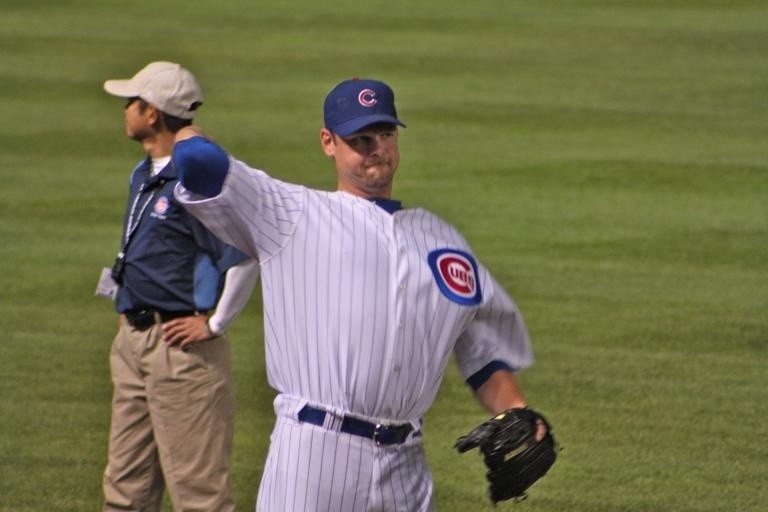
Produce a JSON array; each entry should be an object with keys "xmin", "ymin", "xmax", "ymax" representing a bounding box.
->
[
  {"xmin": 172, "ymin": 77, "xmax": 549, "ymax": 511},
  {"xmin": 92, "ymin": 59, "xmax": 260, "ymax": 511}
]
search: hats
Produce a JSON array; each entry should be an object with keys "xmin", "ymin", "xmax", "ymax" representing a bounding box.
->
[
  {"xmin": 103, "ymin": 60, "xmax": 204, "ymax": 122},
  {"xmin": 323, "ymin": 77, "xmax": 407, "ymax": 139}
]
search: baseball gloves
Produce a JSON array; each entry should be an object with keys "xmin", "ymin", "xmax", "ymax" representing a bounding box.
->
[{"xmin": 456, "ymin": 406, "xmax": 556, "ymax": 502}]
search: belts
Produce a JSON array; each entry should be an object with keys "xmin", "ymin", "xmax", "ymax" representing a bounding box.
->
[
  {"xmin": 125, "ymin": 312, "xmax": 195, "ymax": 330},
  {"xmin": 300, "ymin": 407, "xmax": 411, "ymax": 445}
]
